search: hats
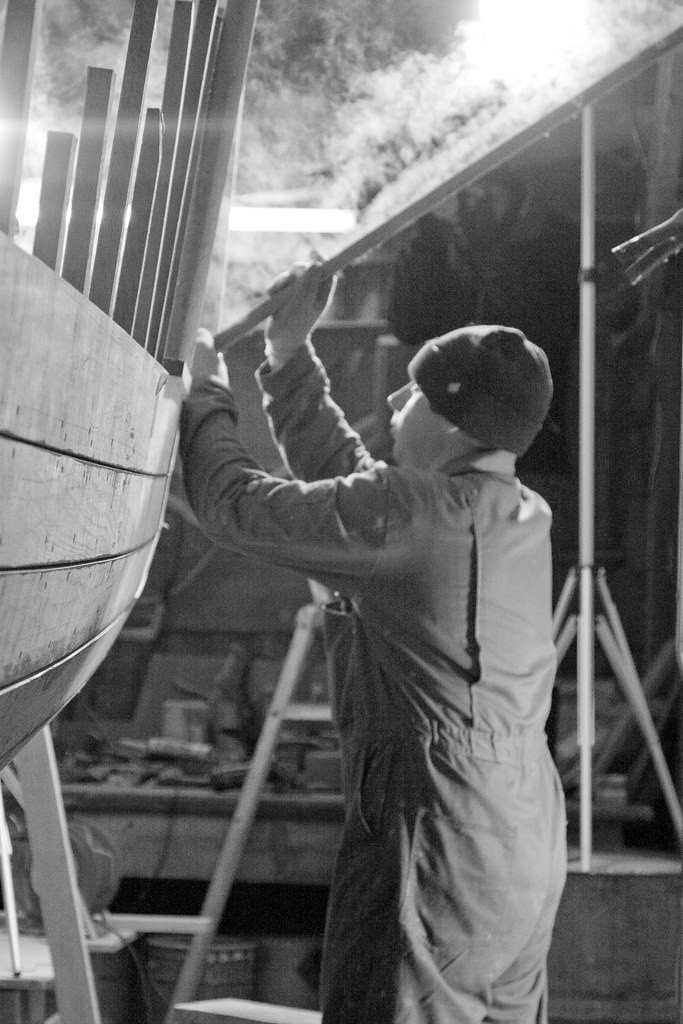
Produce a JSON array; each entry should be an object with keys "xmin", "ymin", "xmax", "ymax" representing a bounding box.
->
[{"xmin": 407, "ymin": 325, "xmax": 553, "ymax": 456}]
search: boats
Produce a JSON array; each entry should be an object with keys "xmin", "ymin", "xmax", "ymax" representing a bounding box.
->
[{"xmin": 1, "ymin": 0, "xmax": 263, "ymax": 764}]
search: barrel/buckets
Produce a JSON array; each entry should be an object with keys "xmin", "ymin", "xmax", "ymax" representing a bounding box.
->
[{"xmin": 143, "ymin": 935, "xmax": 323, "ymax": 1019}]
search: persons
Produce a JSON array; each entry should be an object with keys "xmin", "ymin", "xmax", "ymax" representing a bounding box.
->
[
  {"xmin": 385, "ymin": 163, "xmax": 638, "ymax": 512},
  {"xmin": 179, "ymin": 262, "xmax": 566, "ymax": 1024}
]
